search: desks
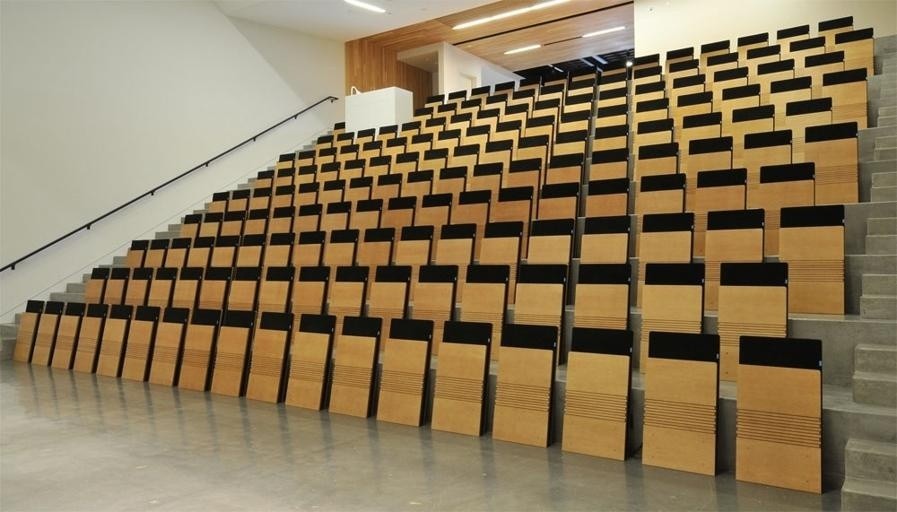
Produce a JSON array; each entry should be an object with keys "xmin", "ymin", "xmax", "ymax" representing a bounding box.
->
[{"xmin": 344, "ymin": 87, "xmax": 414, "ymax": 144}]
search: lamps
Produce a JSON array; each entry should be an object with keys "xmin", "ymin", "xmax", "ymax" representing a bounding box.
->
[{"xmin": 351, "ymin": 87, "xmax": 360, "ymax": 96}]
